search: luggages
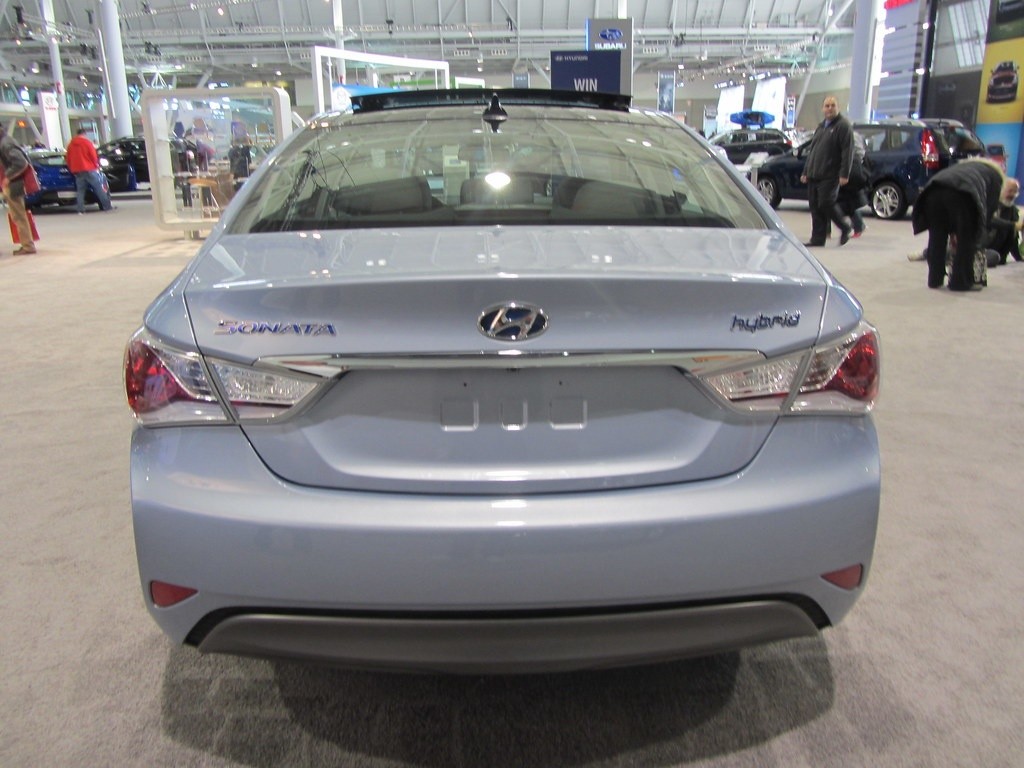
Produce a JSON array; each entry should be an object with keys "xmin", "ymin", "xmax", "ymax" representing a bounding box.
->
[{"xmin": 947, "ymin": 252, "xmax": 987, "ymax": 288}]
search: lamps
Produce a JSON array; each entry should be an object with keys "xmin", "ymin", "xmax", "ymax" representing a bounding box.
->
[
  {"xmin": 80, "ymin": 44, "xmax": 98, "ymax": 59},
  {"xmin": 145, "ymin": 41, "xmax": 163, "ymax": 56}
]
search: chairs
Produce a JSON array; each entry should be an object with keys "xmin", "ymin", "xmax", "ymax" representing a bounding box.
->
[
  {"xmin": 880, "ymin": 132, "xmax": 971, "ymax": 149},
  {"xmin": 304, "ymin": 168, "xmax": 676, "ymax": 224}
]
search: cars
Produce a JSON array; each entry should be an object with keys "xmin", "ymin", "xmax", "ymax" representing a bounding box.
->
[
  {"xmin": 11, "ymin": 134, "xmax": 216, "ymax": 212},
  {"xmin": 120, "ymin": 78, "xmax": 887, "ymax": 664},
  {"xmin": 703, "ymin": 109, "xmax": 989, "ymax": 220}
]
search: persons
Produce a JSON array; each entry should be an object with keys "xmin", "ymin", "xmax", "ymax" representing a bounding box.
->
[
  {"xmin": 35, "ymin": 138, "xmax": 46, "ymax": 148},
  {"xmin": 912, "ymin": 158, "xmax": 1003, "ymax": 290},
  {"xmin": 800, "ymin": 95, "xmax": 854, "ymax": 247},
  {"xmin": 0, "ymin": 123, "xmax": 37, "ymax": 255},
  {"xmin": 66, "ymin": 129, "xmax": 117, "ymax": 215},
  {"xmin": 827, "ymin": 132, "xmax": 867, "ymax": 239},
  {"xmin": 908, "ymin": 177, "xmax": 1024, "ymax": 265}
]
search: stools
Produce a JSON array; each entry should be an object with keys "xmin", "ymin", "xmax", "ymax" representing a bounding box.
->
[{"xmin": 188, "ymin": 178, "xmax": 226, "ymax": 239}]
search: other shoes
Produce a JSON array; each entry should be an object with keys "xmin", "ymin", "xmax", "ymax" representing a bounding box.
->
[
  {"xmin": 907, "ymin": 251, "xmax": 926, "ymax": 262},
  {"xmin": 838, "ymin": 227, "xmax": 854, "ymax": 247},
  {"xmin": 12, "ymin": 247, "xmax": 38, "ymax": 255},
  {"xmin": 78, "ymin": 210, "xmax": 87, "ymax": 216},
  {"xmin": 106, "ymin": 206, "xmax": 119, "ymax": 213},
  {"xmin": 804, "ymin": 242, "xmax": 824, "ymax": 247},
  {"xmin": 852, "ymin": 224, "xmax": 868, "ymax": 240}
]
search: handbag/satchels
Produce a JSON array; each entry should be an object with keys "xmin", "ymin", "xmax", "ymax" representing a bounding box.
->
[{"xmin": 7, "ymin": 210, "xmax": 40, "ymax": 244}]
